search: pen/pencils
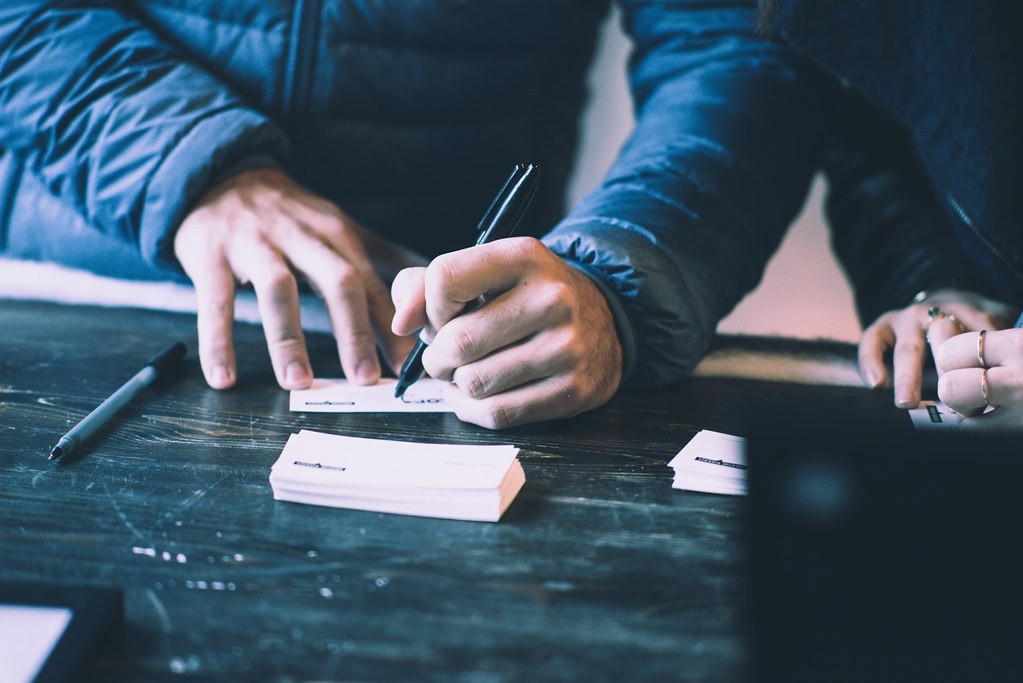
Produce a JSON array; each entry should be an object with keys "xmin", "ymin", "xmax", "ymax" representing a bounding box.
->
[
  {"xmin": 47, "ymin": 340, "xmax": 188, "ymax": 461},
  {"xmin": 392, "ymin": 160, "xmax": 546, "ymax": 403}
]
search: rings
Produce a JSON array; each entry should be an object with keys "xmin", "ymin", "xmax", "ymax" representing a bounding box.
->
[
  {"xmin": 978, "ymin": 329, "xmax": 989, "ymax": 368},
  {"xmin": 980, "ymin": 369, "xmax": 990, "ymax": 405},
  {"xmin": 921, "ymin": 305, "xmax": 967, "ymax": 343}
]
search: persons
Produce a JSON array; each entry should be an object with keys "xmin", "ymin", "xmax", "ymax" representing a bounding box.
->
[
  {"xmin": 793, "ymin": 0, "xmax": 1023, "ymax": 428},
  {"xmin": 3, "ymin": 3, "xmax": 818, "ymax": 433}
]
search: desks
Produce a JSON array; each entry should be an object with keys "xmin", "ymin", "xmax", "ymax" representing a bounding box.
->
[{"xmin": 0, "ymin": 299, "xmax": 1023, "ymax": 682}]
